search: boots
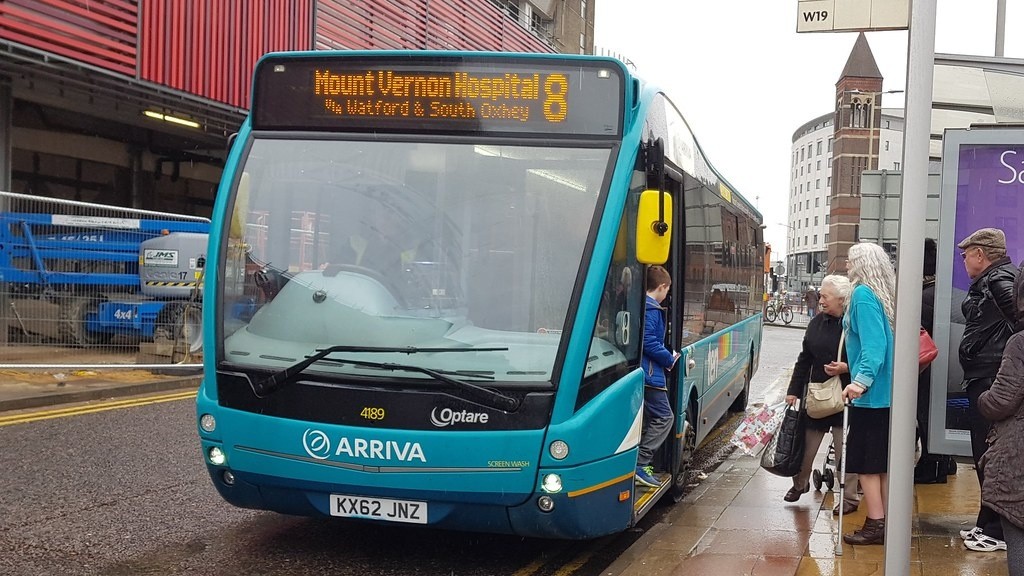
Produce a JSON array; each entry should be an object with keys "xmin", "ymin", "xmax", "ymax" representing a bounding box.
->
[{"xmin": 844, "ymin": 516, "xmax": 885, "ymax": 545}]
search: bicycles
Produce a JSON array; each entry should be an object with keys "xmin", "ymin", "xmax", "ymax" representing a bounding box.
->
[{"xmin": 765, "ymin": 301, "xmax": 793, "ymax": 325}]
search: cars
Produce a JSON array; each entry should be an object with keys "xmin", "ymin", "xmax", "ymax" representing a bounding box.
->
[
  {"xmin": 778, "ymin": 292, "xmax": 804, "ymax": 305},
  {"xmin": 773, "ymin": 290, "xmax": 786, "ymax": 300}
]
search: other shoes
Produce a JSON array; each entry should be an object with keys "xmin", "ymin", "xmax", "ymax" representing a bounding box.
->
[{"xmin": 914, "ymin": 458, "xmax": 947, "ymax": 484}]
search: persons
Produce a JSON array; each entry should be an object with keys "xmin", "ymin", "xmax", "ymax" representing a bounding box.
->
[
  {"xmin": 957, "ymin": 228, "xmax": 1024, "ymax": 551},
  {"xmin": 634, "ymin": 266, "xmax": 676, "ymax": 488},
  {"xmin": 839, "ymin": 242, "xmax": 896, "ymax": 545},
  {"xmin": 914, "ymin": 238, "xmax": 957, "ymax": 484},
  {"xmin": 977, "ymin": 260, "xmax": 1024, "ymax": 576},
  {"xmin": 784, "ymin": 275, "xmax": 860, "ymax": 515},
  {"xmin": 807, "ymin": 287, "xmax": 818, "ymax": 320}
]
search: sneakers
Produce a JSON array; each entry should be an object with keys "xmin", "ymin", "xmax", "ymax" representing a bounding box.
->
[
  {"xmin": 634, "ymin": 465, "xmax": 662, "ymax": 488},
  {"xmin": 963, "ymin": 533, "xmax": 1008, "ymax": 551},
  {"xmin": 959, "ymin": 527, "xmax": 983, "ymax": 539}
]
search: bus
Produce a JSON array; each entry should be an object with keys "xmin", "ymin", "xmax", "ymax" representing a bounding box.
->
[{"xmin": 196, "ymin": 52, "xmax": 764, "ymax": 539}]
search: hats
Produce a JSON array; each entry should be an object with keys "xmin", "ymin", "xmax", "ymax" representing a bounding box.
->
[{"xmin": 956, "ymin": 228, "xmax": 1006, "ymax": 249}]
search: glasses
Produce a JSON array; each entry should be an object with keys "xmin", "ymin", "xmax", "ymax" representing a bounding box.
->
[{"xmin": 959, "ymin": 247, "xmax": 985, "ymax": 258}]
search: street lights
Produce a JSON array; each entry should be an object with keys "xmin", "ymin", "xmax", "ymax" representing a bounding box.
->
[{"xmin": 779, "ymin": 223, "xmax": 795, "ymax": 292}]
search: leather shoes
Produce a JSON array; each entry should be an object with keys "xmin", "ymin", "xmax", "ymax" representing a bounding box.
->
[
  {"xmin": 784, "ymin": 482, "xmax": 809, "ymax": 501},
  {"xmin": 832, "ymin": 500, "xmax": 858, "ymax": 515}
]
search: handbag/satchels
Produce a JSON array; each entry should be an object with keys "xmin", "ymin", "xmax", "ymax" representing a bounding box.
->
[
  {"xmin": 805, "ymin": 377, "xmax": 845, "ymax": 419},
  {"xmin": 917, "ymin": 323, "xmax": 939, "ymax": 367},
  {"xmin": 814, "ymin": 306, "xmax": 821, "ymax": 316},
  {"xmin": 728, "ymin": 400, "xmax": 807, "ymax": 478}
]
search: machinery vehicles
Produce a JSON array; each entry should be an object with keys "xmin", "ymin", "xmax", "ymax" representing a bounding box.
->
[{"xmin": 1, "ymin": 211, "xmax": 260, "ymax": 355}]
